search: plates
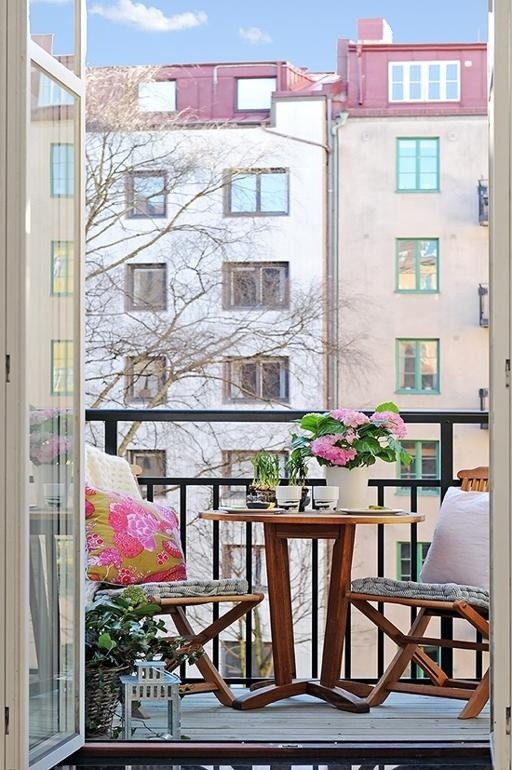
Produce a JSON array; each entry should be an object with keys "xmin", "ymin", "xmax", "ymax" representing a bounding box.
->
[
  {"xmin": 217, "ymin": 506, "xmax": 286, "ymax": 514},
  {"xmin": 247, "ymin": 503, "xmax": 270, "ymax": 509},
  {"xmin": 339, "ymin": 507, "xmax": 401, "ymax": 514}
]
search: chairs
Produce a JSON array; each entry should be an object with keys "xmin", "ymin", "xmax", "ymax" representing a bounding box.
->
[
  {"xmin": 79, "ymin": 445, "xmax": 264, "ymax": 708},
  {"xmin": 346, "ymin": 466, "xmax": 490, "ymax": 720}
]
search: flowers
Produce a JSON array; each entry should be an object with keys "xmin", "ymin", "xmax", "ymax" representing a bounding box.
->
[
  {"xmin": 26, "ymin": 404, "xmax": 73, "ymax": 465},
  {"xmin": 290, "ymin": 402, "xmax": 418, "ymax": 469}
]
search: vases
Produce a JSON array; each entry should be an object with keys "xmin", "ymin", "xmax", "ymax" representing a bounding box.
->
[
  {"xmin": 30, "ymin": 463, "xmax": 71, "ymax": 508},
  {"xmin": 323, "ymin": 464, "xmax": 369, "ymax": 508}
]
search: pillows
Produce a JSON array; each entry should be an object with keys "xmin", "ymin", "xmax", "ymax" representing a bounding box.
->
[
  {"xmin": 84, "ymin": 484, "xmax": 187, "ymax": 585},
  {"xmin": 419, "ymin": 488, "xmax": 490, "ymax": 594}
]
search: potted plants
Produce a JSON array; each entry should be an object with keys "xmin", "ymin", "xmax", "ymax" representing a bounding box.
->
[
  {"xmin": 247, "ymin": 451, "xmax": 277, "ymax": 508},
  {"xmin": 287, "ymin": 444, "xmax": 313, "ymax": 513},
  {"xmin": 84, "ymin": 591, "xmax": 204, "ymax": 737}
]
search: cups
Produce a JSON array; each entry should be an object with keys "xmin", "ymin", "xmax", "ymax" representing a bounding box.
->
[
  {"xmin": 275, "ymin": 486, "xmax": 303, "ymax": 513},
  {"xmin": 312, "ymin": 486, "xmax": 340, "ymax": 514}
]
